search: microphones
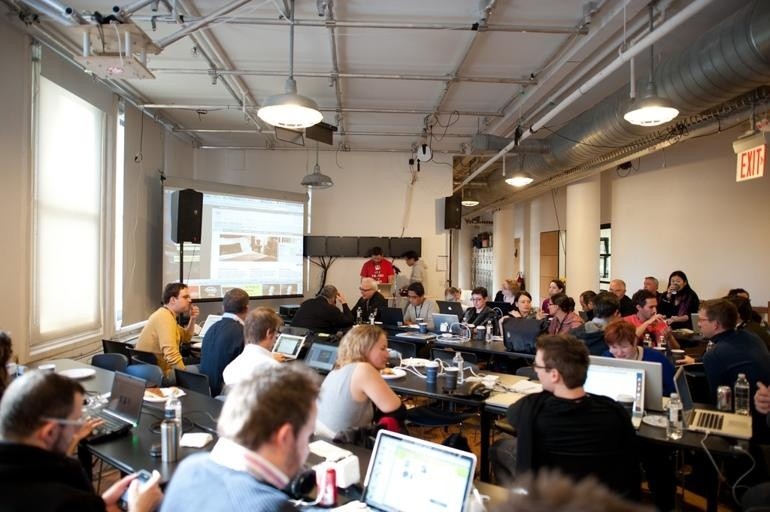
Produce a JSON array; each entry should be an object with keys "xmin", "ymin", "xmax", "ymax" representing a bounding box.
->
[{"xmin": 392, "ymin": 264, "xmax": 401, "ymax": 273}]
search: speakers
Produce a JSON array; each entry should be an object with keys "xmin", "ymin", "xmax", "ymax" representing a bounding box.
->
[
  {"xmin": 303, "ymin": 235, "xmax": 422, "ymax": 258},
  {"xmin": 445, "ymin": 195, "xmax": 460, "ymax": 230},
  {"xmin": 171, "ymin": 189, "xmax": 205, "ymax": 244}
]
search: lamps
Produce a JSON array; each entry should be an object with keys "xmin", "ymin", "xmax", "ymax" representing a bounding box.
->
[
  {"xmin": 622, "ymin": 1, "xmax": 680, "ymax": 127},
  {"xmin": 505, "ymin": 154, "xmax": 533, "ymax": 186},
  {"xmin": 257, "ymin": 0, "xmax": 322, "ymax": 130},
  {"xmin": 301, "ymin": 142, "xmax": 334, "ymax": 188},
  {"xmin": 460, "ymin": 161, "xmax": 479, "ymax": 207}
]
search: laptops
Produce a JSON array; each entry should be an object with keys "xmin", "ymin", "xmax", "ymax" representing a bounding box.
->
[
  {"xmin": 436, "ymin": 300, "xmax": 464, "ymax": 321},
  {"xmin": 304, "ymin": 340, "xmax": 340, "ymax": 374},
  {"xmin": 271, "ymin": 332, "xmax": 306, "ymax": 363},
  {"xmin": 85, "ymin": 370, "xmax": 147, "ymax": 444},
  {"xmin": 673, "ymin": 365, "xmax": 753, "ymax": 440},
  {"xmin": 371, "ymin": 306, "xmax": 408, "ymax": 326},
  {"xmin": 588, "ymin": 355, "xmax": 672, "ymax": 411},
  {"xmin": 191, "ymin": 314, "xmax": 223, "ymax": 342},
  {"xmin": 691, "ymin": 313, "xmax": 702, "ymax": 334},
  {"xmin": 583, "ymin": 362, "xmax": 647, "ymax": 430},
  {"xmin": 334, "ymin": 429, "xmax": 477, "ymax": 512},
  {"xmin": 432, "ymin": 313, "xmax": 460, "ymax": 333},
  {"xmin": 486, "ymin": 301, "xmax": 510, "ymax": 318}
]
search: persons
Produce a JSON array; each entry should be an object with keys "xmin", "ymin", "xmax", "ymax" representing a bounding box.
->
[
  {"xmin": 360, "ymin": 246, "xmax": 395, "ymax": 283},
  {"xmin": 1, "ymin": 271, "xmax": 769, "ymax": 512},
  {"xmin": 402, "ymin": 250, "xmax": 428, "ymax": 296}
]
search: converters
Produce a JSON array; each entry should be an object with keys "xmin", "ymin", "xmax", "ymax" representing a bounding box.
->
[{"xmin": 442, "ymin": 333, "xmax": 452, "ymax": 338}]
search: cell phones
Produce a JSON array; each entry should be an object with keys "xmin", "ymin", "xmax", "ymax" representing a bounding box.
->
[{"xmin": 118, "ymin": 469, "xmax": 153, "ymax": 511}]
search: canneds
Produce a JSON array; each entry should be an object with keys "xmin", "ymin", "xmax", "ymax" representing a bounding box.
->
[
  {"xmin": 316, "ymin": 467, "xmax": 337, "ymax": 508},
  {"xmin": 716, "ymin": 385, "xmax": 732, "ymax": 411}
]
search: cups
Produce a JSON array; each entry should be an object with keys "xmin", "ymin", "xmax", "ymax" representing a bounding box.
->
[
  {"xmin": 670, "ymin": 283, "xmax": 681, "ymax": 295},
  {"xmin": 416, "ymin": 317, "xmax": 496, "ymax": 344},
  {"xmin": 542, "ymin": 313, "xmax": 549, "ymax": 321},
  {"xmin": 425, "ymin": 360, "xmax": 438, "ymax": 384},
  {"xmin": 38, "ymin": 365, "xmax": 56, "ymax": 375},
  {"xmin": 444, "ymin": 365, "xmax": 461, "ymax": 391}
]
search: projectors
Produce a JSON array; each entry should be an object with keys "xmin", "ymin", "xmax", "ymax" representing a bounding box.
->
[{"xmin": 279, "ymin": 304, "xmax": 302, "ymax": 315}]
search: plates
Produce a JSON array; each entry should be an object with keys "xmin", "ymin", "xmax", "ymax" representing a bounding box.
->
[
  {"xmin": 402, "ymin": 357, "xmax": 430, "ymax": 368},
  {"xmin": 57, "ymin": 366, "xmax": 96, "ymax": 382},
  {"xmin": 379, "ymin": 366, "xmax": 407, "ymax": 380},
  {"xmin": 643, "ymin": 413, "xmax": 670, "ymax": 430}
]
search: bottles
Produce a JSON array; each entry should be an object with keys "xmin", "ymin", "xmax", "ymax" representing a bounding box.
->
[
  {"xmin": 642, "ymin": 312, "xmax": 687, "ymax": 369},
  {"xmin": 473, "ymin": 230, "xmax": 493, "ymax": 249},
  {"xmin": 452, "ymin": 351, "xmax": 465, "ymax": 386},
  {"xmin": 733, "ymin": 373, "xmax": 751, "ymax": 416},
  {"xmin": 356, "ymin": 306, "xmax": 375, "ymax": 326},
  {"xmin": 665, "ymin": 392, "xmax": 685, "ymax": 440},
  {"xmin": 164, "ymin": 392, "xmax": 183, "ymax": 442},
  {"xmin": 160, "ymin": 419, "xmax": 180, "ymax": 464}
]
